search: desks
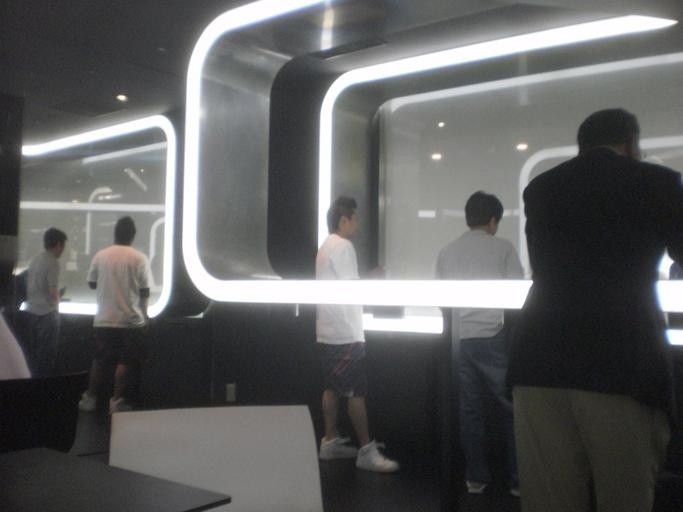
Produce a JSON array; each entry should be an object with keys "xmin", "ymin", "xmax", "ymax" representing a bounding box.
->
[{"xmin": 0, "ymin": 442, "xmax": 235, "ymax": 510}]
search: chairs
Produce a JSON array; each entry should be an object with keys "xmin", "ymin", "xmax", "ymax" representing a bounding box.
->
[{"xmin": 107, "ymin": 401, "xmax": 331, "ymax": 508}]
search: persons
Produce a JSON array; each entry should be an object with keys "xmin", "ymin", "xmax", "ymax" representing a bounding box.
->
[
  {"xmin": 500, "ymin": 103, "xmax": 682, "ymax": 509},
  {"xmin": 312, "ymin": 193, "xmax": 402, "ymax": 476},
  {"xmin": 430, "ymin": 186, "xmax": 531, "ymax": 500},
  {"xmin": 24, "ymin": 226, "xmax": 72, "ymax": 378},
  {"xmin": 76, "ymin": 214, "xmax": 153, "ymax": 417}
]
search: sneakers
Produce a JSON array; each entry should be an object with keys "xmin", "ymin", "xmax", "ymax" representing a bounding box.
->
[
  {"xmin": 78, "ymin": 390, "xmax": 98, "ymax": 412},
  {"xmin": 355, "ymin": 437, "xmax": 400, "ymax": 475},
  {"xmin": 319, "ymin": 434, "xmax": 357, "ymax": 462},
  {"xmin": 108, "ymin": 395, "xmax": 134, "ymax": 414}
]
